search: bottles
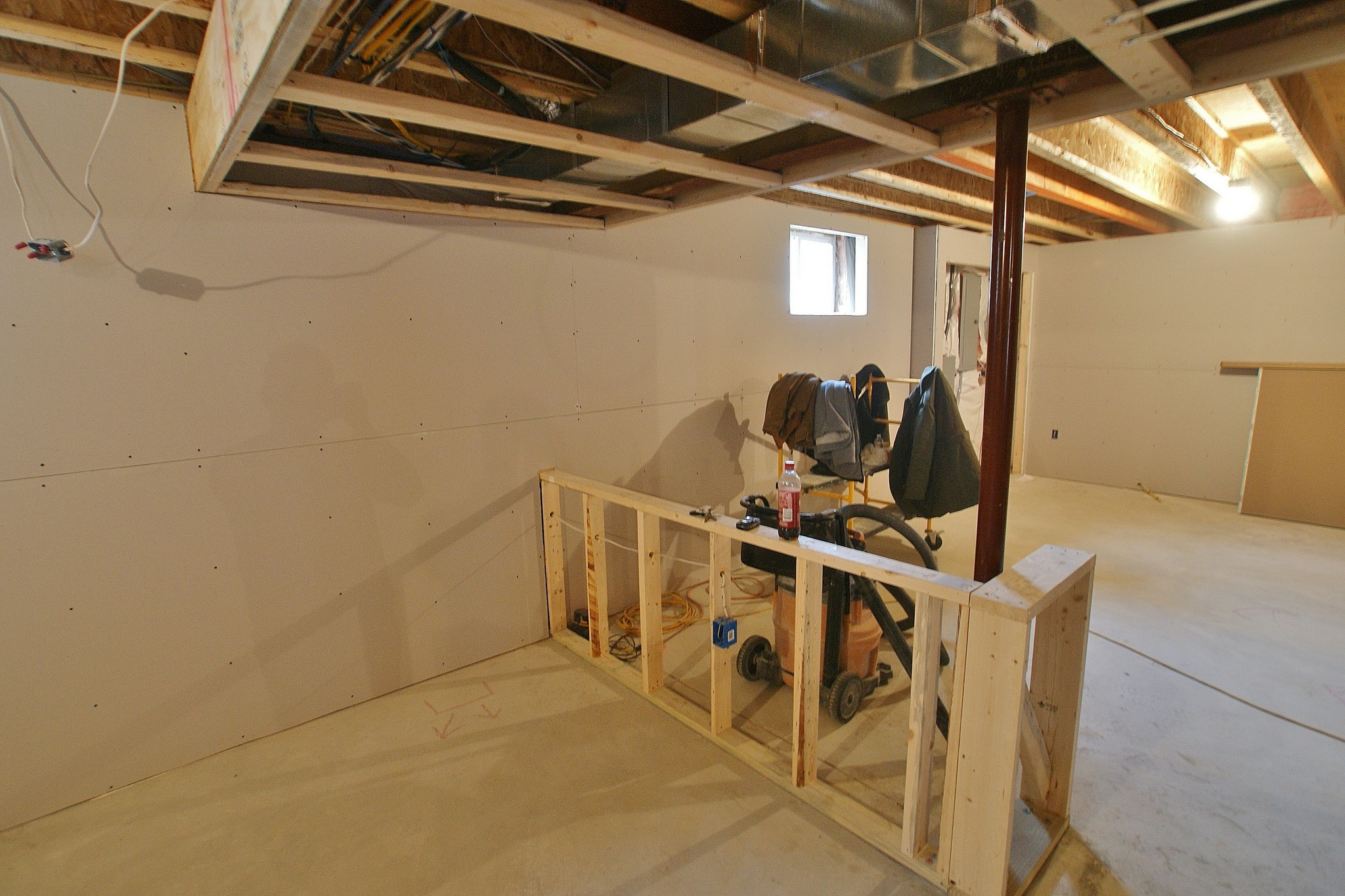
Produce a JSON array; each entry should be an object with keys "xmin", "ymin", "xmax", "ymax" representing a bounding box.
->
[{"xmin": 778, "ymin": 460, "xmax": 802, "ymax": 542}]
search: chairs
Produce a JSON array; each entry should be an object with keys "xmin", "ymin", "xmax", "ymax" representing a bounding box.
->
[{"xmin": 741, "ymin": 494, "xmax": 868, "ymax": 582}]
